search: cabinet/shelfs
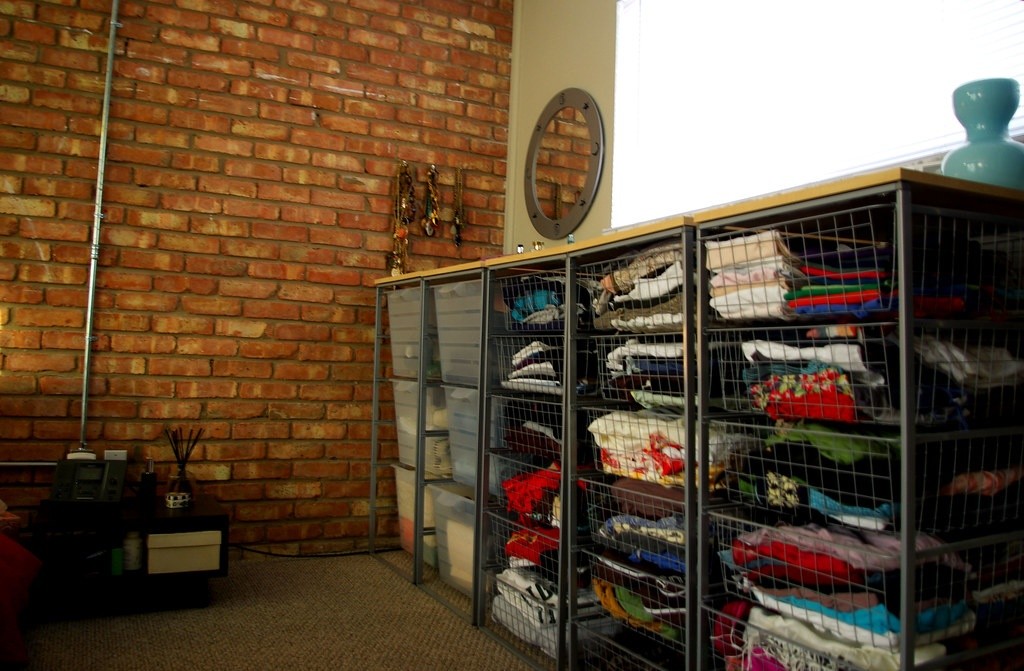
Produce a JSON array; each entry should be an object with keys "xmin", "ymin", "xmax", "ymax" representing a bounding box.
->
[{"xmin": 368, "ymin": 167, "xmax": 1024, "ymax": 671}]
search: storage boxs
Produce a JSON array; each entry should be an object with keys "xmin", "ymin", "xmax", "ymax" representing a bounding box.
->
[
  {"xmin": 383, "ymin": 280, "xmax": 482, "ymax": 599},
  {"xmin": 147, "ymin": 530, "xmax": 222, "ymax": 574}
]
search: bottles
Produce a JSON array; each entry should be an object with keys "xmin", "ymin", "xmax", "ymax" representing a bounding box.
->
[
  {"xmin": 140, "ymin": 459, "xmax": 157, "ymax": 507},
  {"xmin": 122, "ymin": 533, "xmax": 142, "ymax": 570}
]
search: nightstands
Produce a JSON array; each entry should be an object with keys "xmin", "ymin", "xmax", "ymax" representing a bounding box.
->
[{"xmin": 29, "ymin": 493, "xmax": 229, "ymax": 609}]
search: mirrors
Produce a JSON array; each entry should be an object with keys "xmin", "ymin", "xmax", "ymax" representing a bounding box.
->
[{"xmin": 524, "ymin": 88, "xmax": 604, "ymax": 240}]
